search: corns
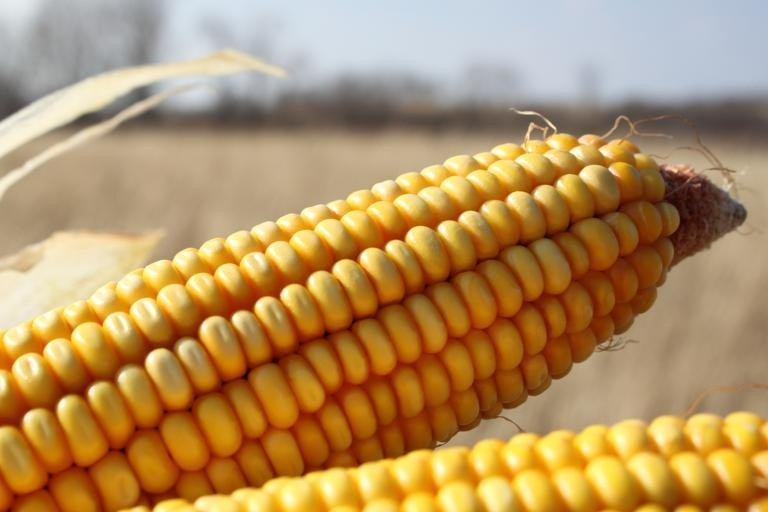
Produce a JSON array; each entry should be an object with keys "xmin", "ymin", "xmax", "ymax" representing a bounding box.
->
[
  {"xmin": 1, "ymin": 134, "xmax": 746, "ymax": 512},
  {"xmin": 121, "ymin": 412, "xmax": 768, "ymax": 512}
]
